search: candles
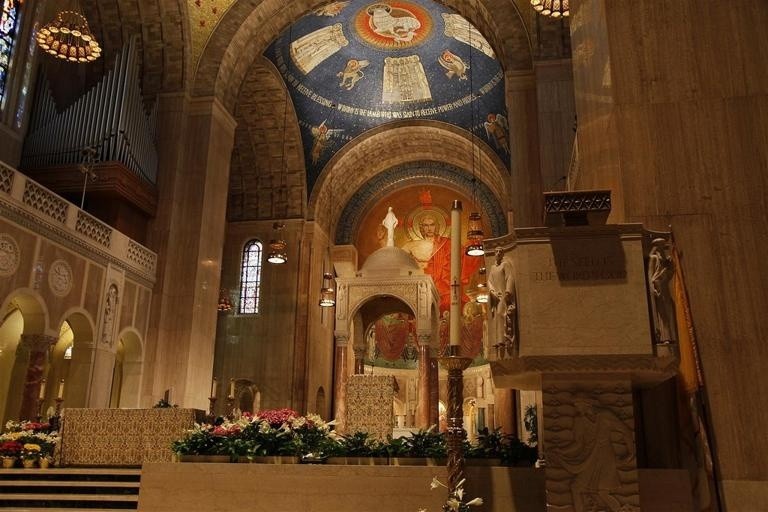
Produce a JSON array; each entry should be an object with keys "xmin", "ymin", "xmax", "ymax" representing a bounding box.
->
[
  {"xmin": 58, "ymin": 383, "xmax": 64, "ymax": 399},
  {"xmin": 230, "ymin": 381, "xmax": 235, "ymax": 399},
  {"xmin": 39, "ymin": 382, "xmax": 45, "ymax": 399},
  {"xmin": 212, "ymin": 381, "xmax": 217, "ymax": 398}
]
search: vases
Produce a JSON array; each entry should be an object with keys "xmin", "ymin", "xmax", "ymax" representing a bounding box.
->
[{"xmin": 2, "ymin": 457, "xmax": 50, "ymax": 469}]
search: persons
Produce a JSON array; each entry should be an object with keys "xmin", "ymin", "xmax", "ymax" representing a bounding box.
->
[
  {"xmin": 400, "ymin": 215, "xmax": 481, "ymax": 320},
  {"xmin": 487, "ymin": 247, "xmax": 514, "ymax": 349},
  {"xmin": 550, "ymin": 392, "xmax": 634, "ymax": 512},
  {"xmin": 648, "ymin": 238, "xmax": 674, "ymax": 346},
  {"xmin": 102, "ymin": 289, "xmax": 117, "ymax": 344}
]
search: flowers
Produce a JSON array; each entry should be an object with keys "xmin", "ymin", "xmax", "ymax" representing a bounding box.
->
[
  {"xmin": 0, "ymin": 418, "xmax": 58, "ymax": 461},
  {"xmin": 419, "ymin": 477, "xmax": 485, "ymax": 512},
  {"xmin": 171, "ymin": 405, "xmax": 389, "ymax": 461}
]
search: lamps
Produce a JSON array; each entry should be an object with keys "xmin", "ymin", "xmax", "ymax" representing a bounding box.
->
[
  {"xmin": 464, "ymin": 21, "xmax": 491, "ymax": 303},
  {"xmin": 37, "ymin": 0, "xmax": 103, "ymax": 65},
  {"xmin": 268, "ymin": 16, "xmax": 339, "ymax": 310}
]
